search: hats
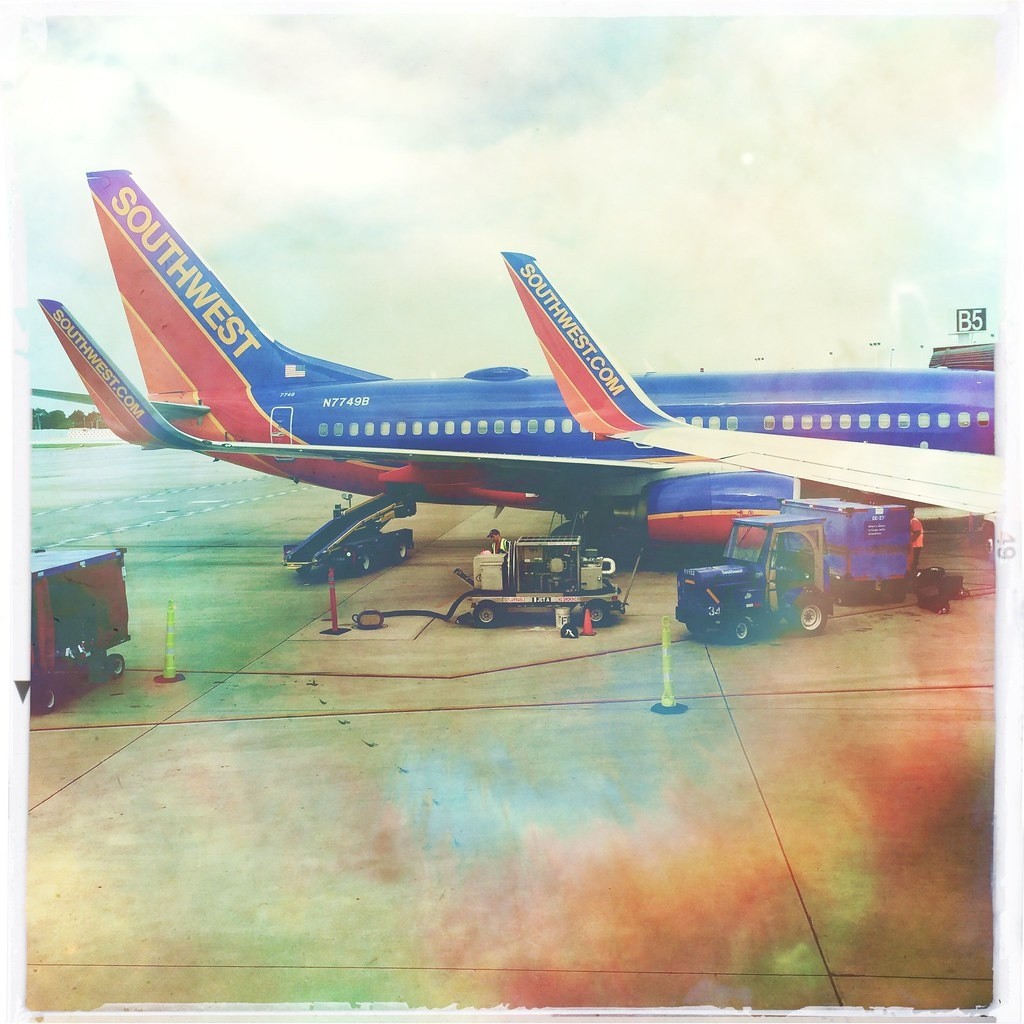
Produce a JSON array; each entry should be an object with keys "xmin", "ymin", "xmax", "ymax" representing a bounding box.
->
[{"xmin": 487, "ymin": 529, "xmax": 499, "ymax": 537}]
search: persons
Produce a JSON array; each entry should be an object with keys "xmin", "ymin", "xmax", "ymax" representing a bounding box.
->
[
  {"xmin": 487, "ymin": 529, "xmax": 510, "ymax": 554},
  {"xmin": 909, "ymin": 510, "xmax": 923, "ymax": 571}
]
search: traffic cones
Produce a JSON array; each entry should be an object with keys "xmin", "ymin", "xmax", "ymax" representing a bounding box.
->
[
  {"xmin": 579, "ymin": 608, "xmax": 597, "ymax": 636},
  {"xmin": 154, "ymin": 600, "xmax": 187, "ymax": 683}
]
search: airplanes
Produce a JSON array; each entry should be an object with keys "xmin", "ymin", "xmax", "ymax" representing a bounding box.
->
[{"xmin": 30, "ymin": 169, "xmax": 995, "ymax": 577}]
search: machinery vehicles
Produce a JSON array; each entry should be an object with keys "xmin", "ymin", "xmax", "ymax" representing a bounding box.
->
[{"xmin": 466, "ymin": 537, "xmax": 631, "ymax": 628}]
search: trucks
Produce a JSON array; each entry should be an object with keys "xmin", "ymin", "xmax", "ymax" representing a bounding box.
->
[
  {"xmin": 779, "ymin": 497, "xmax": 911, "ymax": 605},
  {"xmin": 31, "ymin": 548, "xmax": 132, "ymax": 720}
]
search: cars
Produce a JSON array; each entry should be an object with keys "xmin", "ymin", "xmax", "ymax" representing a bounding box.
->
[{"xmin": 672, "ymin": 516, "xmax": 833, "ymax": 644}]
